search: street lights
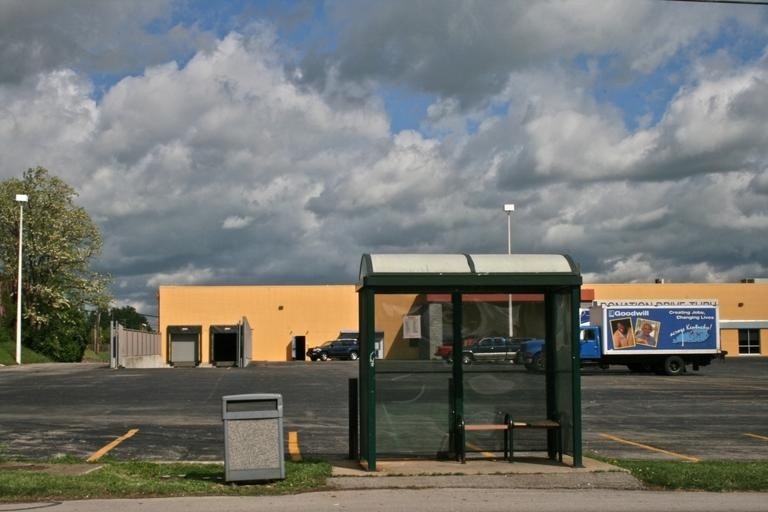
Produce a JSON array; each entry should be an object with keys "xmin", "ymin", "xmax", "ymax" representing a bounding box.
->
[
  {"xmin": 504, "ymin": 204, "xmax": 515, "ymax": 364},
  {"xmin": 15, "ymin": 194, "xmax": 28, "ymax": 365}
]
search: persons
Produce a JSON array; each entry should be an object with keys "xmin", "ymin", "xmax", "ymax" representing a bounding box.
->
[
  {"xmin": 634, "ymin": 323, "xmax": 656, "ymax": 346},
  {"xmin": 612, "ymin": 320, "xmax": 635, "ymax": 349}
]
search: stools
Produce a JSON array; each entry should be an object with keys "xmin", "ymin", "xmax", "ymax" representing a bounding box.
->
[
  {"xmin": 456, "ymin": 415, "xmax": 510, "ymax": 464},
  {"xmin": 506, "ymin": 415, "xmax": 562, "ymax": 463}
]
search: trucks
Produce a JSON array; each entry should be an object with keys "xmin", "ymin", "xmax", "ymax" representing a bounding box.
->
[{"xmin": 520, "ymin": 298, "xmax": 728, "ymax": 376}]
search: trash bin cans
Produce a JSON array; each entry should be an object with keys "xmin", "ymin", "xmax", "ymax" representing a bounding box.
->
[{"xmin": 221, "ymin": 393, "xmax": 285, "ymax": 482}]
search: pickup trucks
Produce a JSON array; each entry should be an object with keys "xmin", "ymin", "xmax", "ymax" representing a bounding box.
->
[{"xmin": 449, "ymin": 337, "xmax": 536, "ymax": 367}]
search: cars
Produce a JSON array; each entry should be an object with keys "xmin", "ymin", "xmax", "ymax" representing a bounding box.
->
[
  {"xmin": 433, "ymin": 334, "xmax": 481, "ymax": 363},
  {"xmin": 306, "ymin": 340, "xmax": 360, "ymax": 361}
]
